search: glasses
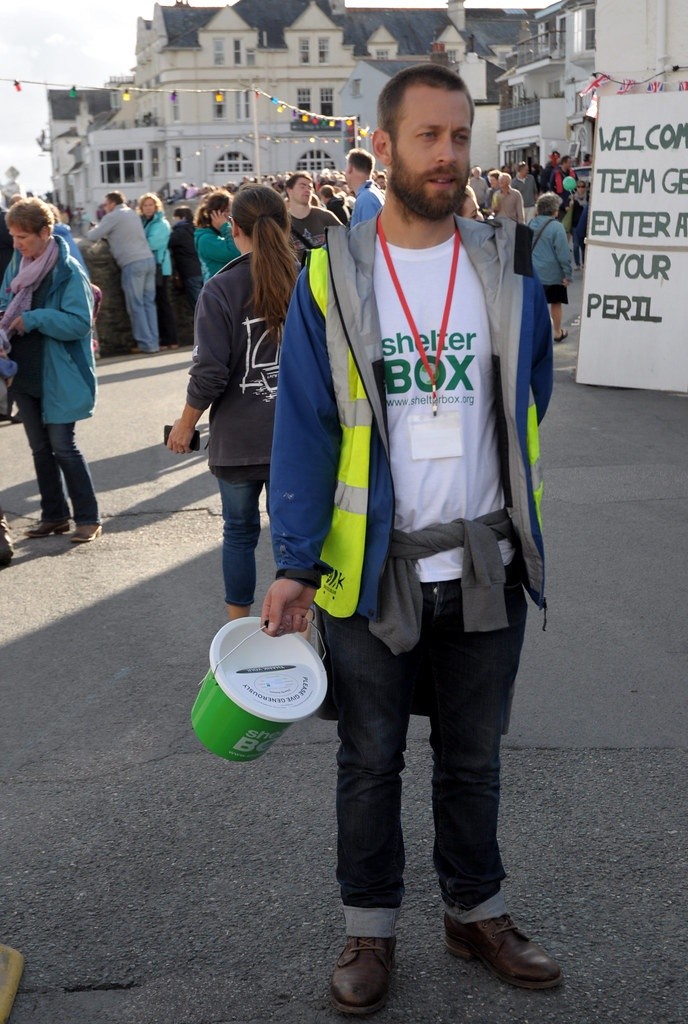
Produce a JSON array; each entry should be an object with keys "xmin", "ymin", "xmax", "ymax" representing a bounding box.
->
[{"xmin": 577, "ymin": 186, "xmax": 584, "ymax": 188}]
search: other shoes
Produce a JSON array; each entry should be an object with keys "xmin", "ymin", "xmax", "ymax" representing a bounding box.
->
[
  {"xmin": 160, "ymin": 346, "xmax": 167, "ymax": 350},
  {"xmin": 574, "ymin": 265, "xmax": 580, "ymax": 271},
  {"xmin": 131, "ymin": 347, "xmax": 155, "ymax": 354},
  {"xmin": 12, "ymin": 410, "xmax": 23, "ymax": 423},
  {"xmin": 0, "ymin": 413, "xmax": 12, "ymax": 421},
  {"xmin": 170, "ymin": 344, "xmax": 178, "ymax": 349}
]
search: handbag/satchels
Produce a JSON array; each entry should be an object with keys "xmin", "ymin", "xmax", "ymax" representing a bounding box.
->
[
  {"xmin": 156, "ymin": 263, "xmax": 163, "ymax": 286},
  {"xmin": 562, "ymin": 204, "xmax": 573, "ymax": 232}
]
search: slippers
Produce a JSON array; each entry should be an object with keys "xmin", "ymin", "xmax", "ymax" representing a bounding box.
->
[{"xmin": 554, "ymin": 329, "xmax": 568, "ymax": 341}]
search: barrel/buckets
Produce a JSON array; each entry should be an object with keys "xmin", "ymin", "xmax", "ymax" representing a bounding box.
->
[{"xmin": 191, "ymin": 617, "xmax": 330, "ymax": 762}]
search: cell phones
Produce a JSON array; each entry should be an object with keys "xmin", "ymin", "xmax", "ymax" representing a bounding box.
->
[{"xmin": 164, "ymin": 425, "xmax": 200, "ymax": 451}]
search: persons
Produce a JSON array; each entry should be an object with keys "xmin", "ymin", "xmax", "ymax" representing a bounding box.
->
[
  {"xmin": 319, "ymin": 185, "xmax": 350, "ymax": 227},
  {"xmin": 344, "ymin": 149, "xmax": 386, "ymax": 230},
  {"xmin": 193, "ymin": 191, "xmax": 241, "ymax": 283},
  {"xmin": 525, "ymin": 192, "xmax": 572, "ymax": 343},
  {"xmin": 0, "ymin": 192, "xmax": 99, "ymax": 571},
  {"xmin": 76, "ymin": 190, "xmax": 159, "ymax": 354},
  {"xmin": 202, "ymin": 164, "xmax": 389, "ymax": 215},
  {"xmin": 136, "ymin": 194, "xmax": 181, "ymax": 349},
  {"xmin": 259, "ymin": 63, "xmax": 563, "ymax": 1010},
  {"xmin": 456, "ymin": 151, "xmax": 593, "ymax": 277},
  {"xmin": 167, "ymin": 184, "xmax": 315, "ymax": 646},
  {"xmin": 95, "ymin": 179, "xmax": 202, "ymax": 221},
  {"xmin": 169, "ymin": 206, "xmax": 205, "ymax": 305},
  {"xmin": 284, "ymin": 172, "xmax": 343, "ymax": 269}
]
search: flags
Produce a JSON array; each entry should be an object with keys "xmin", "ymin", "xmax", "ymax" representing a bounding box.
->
[
  {"xmin": 677, "ymin": 81, "xmax": 688, "ymax": 92},
  {"xmin": 578, "ymin": 74, "xmax": 613, "ymax": 98},
  {"xmin": 645, "ymin": 81, "xmax": 664, "ymax": 93},
  {"xmin": 616, "ymin": 79, "xmax": 635, "ymax": 95}
]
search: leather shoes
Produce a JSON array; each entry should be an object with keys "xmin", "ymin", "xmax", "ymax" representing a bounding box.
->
[
  {"xmin": 24, "ymin": 520, "xmax": 69, "ymax": 536},
  {"xmin": 70, "ymin": 524, "xmax": 102, "ymax": 541},
  {"xmin": 444, "ymin": 911, "xmax": 562, "ymax": 988},
  {"xmin": 329, "ymin": 936, "xmax": 397, "ymax": 1013}
]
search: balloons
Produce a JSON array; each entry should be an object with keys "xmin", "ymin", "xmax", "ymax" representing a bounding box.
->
[{"xmin": 562, "ymin": 175, "xmax": 576, "ymax": 192}]
